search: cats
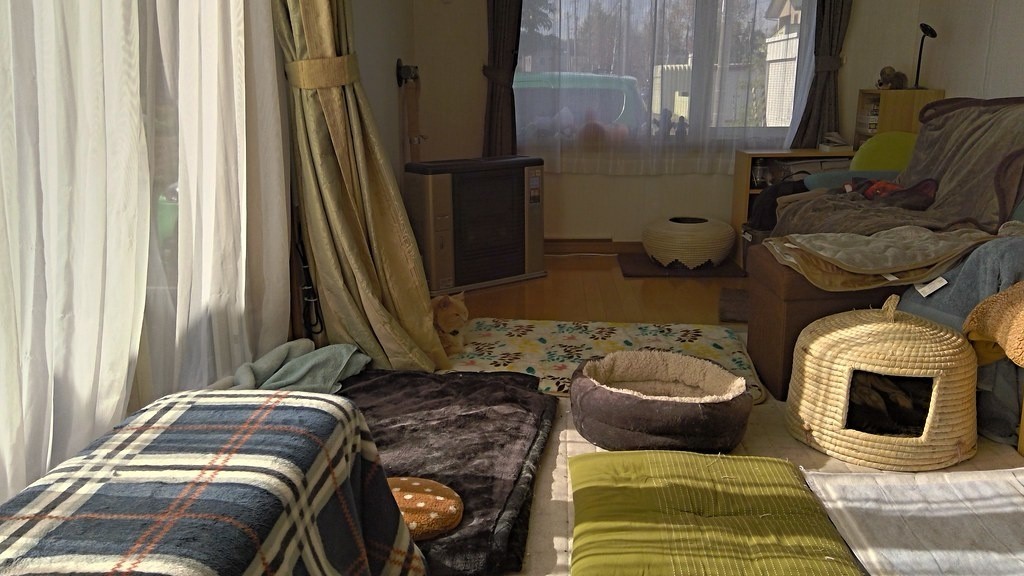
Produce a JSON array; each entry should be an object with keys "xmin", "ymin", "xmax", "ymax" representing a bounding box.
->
[{"xmin": 433, "ymin": 290, "xmax": 468, "ymax": 355}]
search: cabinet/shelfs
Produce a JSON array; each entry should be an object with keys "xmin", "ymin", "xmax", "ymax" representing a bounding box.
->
[
  {"xmin": 731, "ymin": 148, "xmax": 856, "ymax": 270},
  {"xmin": 853, "ymin": 89, "xmax": 945, "ymax": 150}
]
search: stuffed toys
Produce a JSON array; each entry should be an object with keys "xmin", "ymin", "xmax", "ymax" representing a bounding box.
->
[{"xmin": 877, "ymin": 67, "xmax": 900, "ymax": 90}]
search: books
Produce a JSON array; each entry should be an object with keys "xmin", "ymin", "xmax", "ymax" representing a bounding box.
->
[{"xmin": 818, "ymin": 102, "xmax": 878, "ymax": 152}]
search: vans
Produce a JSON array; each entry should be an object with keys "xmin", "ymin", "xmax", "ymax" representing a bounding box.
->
[{"xmin": 513, "ymin": 73, "xmax": 648, "ymax": 135}]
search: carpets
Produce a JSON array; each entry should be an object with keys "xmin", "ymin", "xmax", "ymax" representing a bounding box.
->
[{"xmin": 617, "ymin": 252, "xmax": 747, "ymax": 278}]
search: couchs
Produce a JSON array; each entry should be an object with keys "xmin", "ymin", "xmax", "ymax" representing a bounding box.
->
[
  {"xmin": 750, "ymin": 98, "xmax": 1024, "ymax": 445},
  {"xmin": 0, "ymin": 391, "xmax": 426, "ymax": 576}
]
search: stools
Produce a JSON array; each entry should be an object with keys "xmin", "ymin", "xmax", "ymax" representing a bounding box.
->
[{"xmin": 746, "ymin": 243, "xmax": 892, "ymax": 402}]
